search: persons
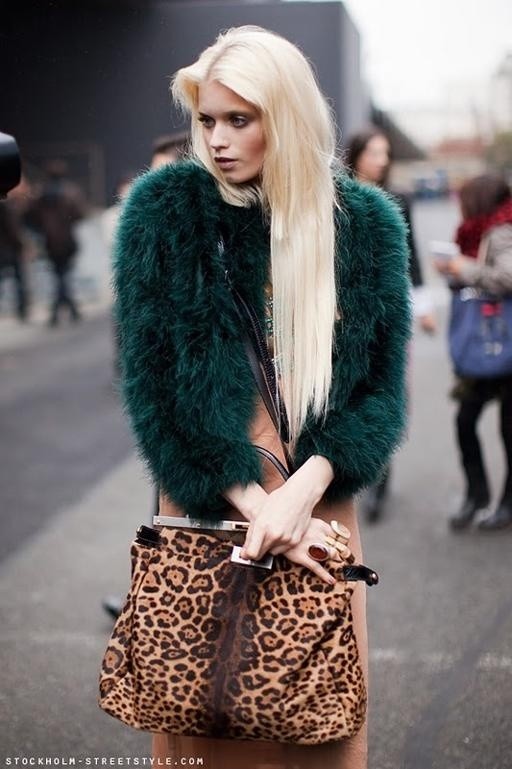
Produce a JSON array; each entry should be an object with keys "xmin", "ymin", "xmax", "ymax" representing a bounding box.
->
[
  {"xmin": 96, "ymin": 23, "xmax": 413, "ymax": 769},
  {"xmin": 1, "ymin": 183, "xmax": 36, "ymax": 319},
  {"xmin": 339, "ymin": 125, "xmax": 438, "ymax": 525},
  {"xmin": 24, "ymin": 155, "xmax": 85, "ymax": 325},
  {"xmin": 424, "ymin": 168, "xmax": 511, "ymax": 532}
]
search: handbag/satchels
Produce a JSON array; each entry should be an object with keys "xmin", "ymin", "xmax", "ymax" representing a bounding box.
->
[
  {"xmin": 447, "ymin": 285, "xmax": 512, "ymax": 382},
  {"xmin": 98, "ymin": 525, "xmax": 379, "ymax": 746}
]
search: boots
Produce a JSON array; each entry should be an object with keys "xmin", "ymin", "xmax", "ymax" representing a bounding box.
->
[{"xmin": 450, "ymin": 480, "xmax": 512, "ymax": 531}]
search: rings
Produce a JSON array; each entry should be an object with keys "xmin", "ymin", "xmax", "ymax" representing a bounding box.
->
[
  {"xmin": 326, "ymin": 534, "xmax": 347, "ymax": 554},
  {"xmin": 330, "ymin": 519, "xmax": 351, "ymax": 541},
  {"xmin": 307, "ymin": 543, "xmax": 330, "ymax": 562}
]
